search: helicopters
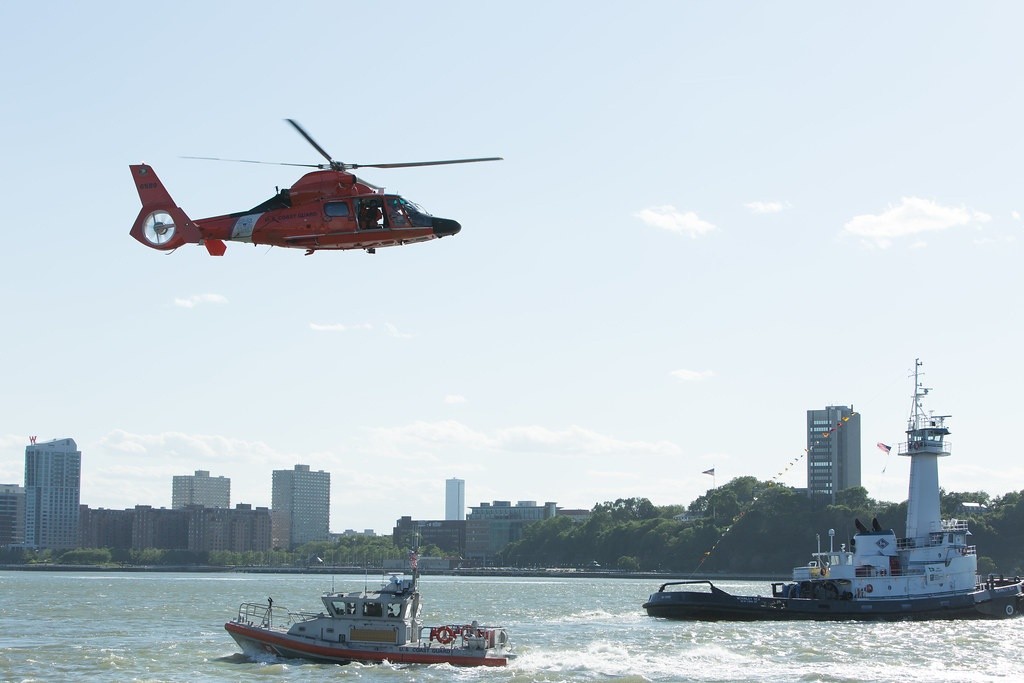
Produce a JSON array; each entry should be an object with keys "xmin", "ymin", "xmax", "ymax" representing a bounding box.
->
[{"xmin": 130, "ymin": 119, "xmax": 504, "ymax": 255}]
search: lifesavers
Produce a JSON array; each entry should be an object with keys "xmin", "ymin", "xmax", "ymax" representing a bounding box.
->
[
  {"xmin": 962, "ymin": 548, "xmax": 967, "ymax": 556},
  {"xmin": 1014, "ymin": 576, "xmax": 1020, "ymax": 583},
  {"xmin": 436, "ymin": 626, "xmax": 454, "ymax": 644},
  {"xmin": 460, "ymin": 625, "xmax": 473, "ymax": 641},
  {"xmin": 845, "ymin": 592, "xmax": 853, "ymax": 601},
  {"xmin": 867, "ymin": 584, "xmax": 873, "ymax": 593}
]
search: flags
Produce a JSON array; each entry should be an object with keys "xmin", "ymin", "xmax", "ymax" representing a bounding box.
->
[
  {"xmin": 702, "ymin": 469, "xmax": 714, "ymax": 476},
  {"xmin": 696, "ymin": 410, "xmax": 855, "ymax": 565},
  {"xmin": 877, "ymin": 442, "xmax": 891, "ymax": 454}
]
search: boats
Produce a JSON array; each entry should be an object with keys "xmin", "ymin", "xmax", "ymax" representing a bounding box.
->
[
  {"xmin": 225, "ymin": 528, "xmax": 515, "ymax": 668},
  {"xmin": 642, "ymin": 359, "xmax": 1024, "ymax": 622}
]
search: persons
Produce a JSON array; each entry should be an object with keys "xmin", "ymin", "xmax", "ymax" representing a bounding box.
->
[{"xmin": 361, "ymin": 200, "xmax": 382, "ymax": 230}]
